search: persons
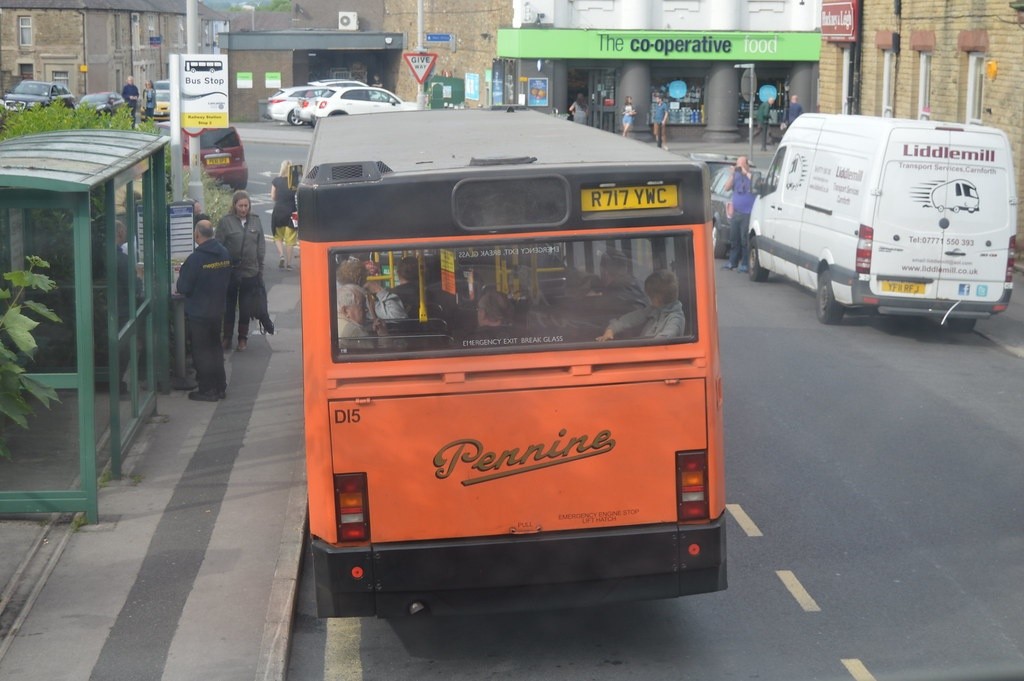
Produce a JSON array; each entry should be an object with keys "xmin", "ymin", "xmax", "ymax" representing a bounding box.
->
[
  {"xmin": 569, "ymin": 93, "xmax": 589, "ymax": 125},
  {"xmin": 654, "ymin": 95, "xmax": 669, "ymax": 151},
  {"xmin": 756, "ymin": 98, "xmax": 775, "ymax": 151},
  {"xmin": 116, "ymin": 218, "xmax": 144, "ymax": 392},
  {"xmin": 143, "ymin": 80, "xmax": 157, "ymax": 123},
  {"xmin": 720, "ymin": 156, "xmax": 757, "ymax": 273},
  {"xmin": 121, "ymin": 76, "xmax": 139, "ymax": 129},
  {"xmin": 369, "ymin": 72, "xmax": 384, "ymax": 88},
  {"xmin": 177, "ymin": 190, "xmax": 266, "ymax": 401},
  {"xmin": 782, "ymin": 95, "xmax": 802, "ymax": 129},
  {"xmin": 620, "ymin": 96, "xmax": 638, "ymax": 137},
  {"xmin": 337, "ymin": 245, "xmax": 686, "ymax": 349},
  {"xmin": 271, "ymin": 160, "xmax": 297, "ymax": 270}
]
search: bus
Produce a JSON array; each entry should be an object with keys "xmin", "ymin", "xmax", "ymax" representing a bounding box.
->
[{"xmin": 285, "ymin": 104, "xmax": 728, "ymax": 619}]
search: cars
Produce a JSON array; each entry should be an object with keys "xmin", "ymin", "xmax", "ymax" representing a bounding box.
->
[
  {"xmin": 139, "ymin": 79, "xmax": 170, "ymax": 122},
  {"xmin": 153, "ymin": 120, "xmax": 248, "ymax": 191},
  {"xmin": 77, "ymin": 91, "xmax": 125, "ymax": 118},
  {"xmin": 266, "ymin": 78, "xmax": 417, "ymax": 131},
  {"xmin": 688, "ymin": 153, "xmax": 771, "ymax": 260}
]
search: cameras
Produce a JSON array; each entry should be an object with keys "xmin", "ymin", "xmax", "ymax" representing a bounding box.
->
[{"xmin": 735, "ymin": 167, "xmax": 742, "ymax": 172}]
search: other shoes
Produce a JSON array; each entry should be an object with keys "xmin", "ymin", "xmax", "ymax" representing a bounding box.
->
[
  {"xmin": 279, "ymin": 256, "xmax": 285, "ymax": 268},
  {"xmin": 237, "ymin": 338, "xmax": 248, "ymax": 351},
  {"xmin": 189, "ymin": 387, "xmax": 219, "ymax": 402},
  {"xmin": 219, "ymin": 389, "xmax": 226, "ymax": 399},
  {"xmin": 724, "ymin": 261, "xmax": 738, "ymax": 268},
  {"xmin": 221, "ymin": 336, "xmax": 232, "ymax": 349},
  {"xmin": 740, "ymin": 263, "xmax": 750, "ymax": 273},
  {"xmin": 287, "ymin": 265, "xmax": 292, "ymax": 271}
]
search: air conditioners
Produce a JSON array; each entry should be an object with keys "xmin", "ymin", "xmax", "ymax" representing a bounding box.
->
[{"xmin": 338, "ymin": 10, "xmax": 360, "ymax": 32}]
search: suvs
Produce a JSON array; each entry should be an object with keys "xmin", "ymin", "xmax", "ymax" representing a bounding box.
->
[{"xmin": 0, "ymin": 77, "xmax": 77, "ymax": 114}]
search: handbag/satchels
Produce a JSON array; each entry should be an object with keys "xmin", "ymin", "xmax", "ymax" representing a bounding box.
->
[{"xmin": 231, "ymin": 259, "xmax": 242, "ymax": 279}]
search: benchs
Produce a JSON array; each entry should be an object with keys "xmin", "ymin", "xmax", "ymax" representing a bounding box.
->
[
  {"xmin": 535, "ymin": 276, "xmax": 577, "ymax": 310},
  {"xmin": 339, "ymin": 303, "xmax": 454, "ymax": 354}
]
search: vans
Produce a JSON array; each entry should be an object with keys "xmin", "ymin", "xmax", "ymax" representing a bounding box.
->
[{"xmin": 746, "ymin": 112, "xmax": 1019, "ymax": 335}]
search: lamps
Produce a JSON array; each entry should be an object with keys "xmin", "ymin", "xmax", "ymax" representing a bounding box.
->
[{"xmin": 295, "ymin": 4, "xmax": 304, "ymax": 15}]
